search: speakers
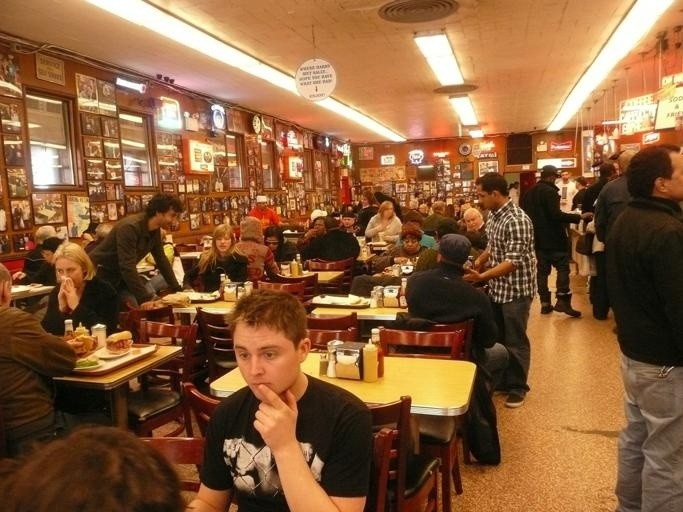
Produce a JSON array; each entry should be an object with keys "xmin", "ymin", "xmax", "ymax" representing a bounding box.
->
[
  {"xmin": 553, "ymin": 290, "xmax": 581, "ymax": 319},
  {"xmin": 539, "ymin": 292, "xmax": 554, "ymax": 315}
]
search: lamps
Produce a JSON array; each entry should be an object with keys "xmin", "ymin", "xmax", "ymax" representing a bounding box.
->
[
  {"xmin": 257, "ymin": 280, "xmax": 304, "ymax": 303},
  {"xmin": 138, "ymin": 436, "xmax": 204, "ymax": 508},
  {"xmin": 306, "ymin": 257, "xmax": 353, "ymax": 296},
  {"xmin": 396, "ymin": 318, "xmax": 474, "ymax": 464},
  {"xmin": 371, "ymin": 396, "xmax": 440, "ymax": 512},
  {"xmin": 197, "ymin": 307, "xmax": 236, "ymax": 382},
  {"xmin": 181, "ymin": 382, "xmax": 221, "ymax": 483},
  {"xmin": 304, "ymin": 325, "xmax": 359, "ymax": 353},
  {"xmin": 275, "ymin": 273, "xmax": 319, "ymax": 299},
  {"xmin": 378, "ymin": 327, "xmax": 466, "ymax": 512},
  {"xmin": 117, "ymin": 305, "xmax": 198, "ymax": 365},
  {"xmin": 129, "ymin": 319, "xmax": 198, "ymax": 440}
]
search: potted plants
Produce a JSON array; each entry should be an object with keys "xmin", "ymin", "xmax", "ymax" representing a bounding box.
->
[
  {"xmin": 13, "ymin": 232, "xmax": 36, "ymax": 252},
  {"xmin": 74, "ymin": 72, "xmax": 98, "ymax": 113},
  {"xmin": 35, "ymin": 52, "xmax": 66, "ymax": 87},
  {"xmin": 80, "ymin": 112, "xmax": 101, "ymax": 136},
  {"xmin": 9, "ymin": 199, "xmax": 35, "ymax": 231},
  {"xmin": 141, "ymin": 195, "xmax": 154, "ymax": 209},
  {"xmin": 66, "ymin": 195, "xmax": 91, "ymax": 237},
  {"xmin": 107, "ymin": 203, "xmax": 117, "ymax": 221},
  {"xmin": 115, "ymin": 182, "xmax": 127, "ymax": 200},
  {"xmin": 399, "ymin": 202, "xmax": 407, "ymax": 207},
  {"xmin": 90, "ymin": 203, "xmax": 107, "ymax": 224},
  {"xmin": 0, "ymin": 234, "xmax": 10, "ymax": 253},
  {"xmin": 2, "ymin": 133, "xmax": 27, "ymax": 166},
  {"xmin": 6, "ymin": 167, "xmax": 32, "ymax": 199},
  {"xmin": 0, "ymin": 46, "xmax": 25, "ymax": 99},
  {"xmin": 30, "ymin": 191, "xmax": 68, "ymax": 226},
  {"xmin": 0, "ymin": 96, "xmax": 27, "ymax": 134},
  {"xmin": 96, "ymin": 79, "xmax": 118, "ymax": 115},
  {"xmin": 100, "ymin": 115, "xmax": 120, "ymax": 137},
  {"xmin": 104, "ymin": 159, "xmax": 123, "ymax": 180},
  {"xmin": 103, "ymin": 137, "xmax": 122, "ymax": 160},
  {"xmin": 358, "ymin": 146, "xmax": 374, "ymax": 160},
  {"xmin": 409, "ymin": 156, "xmax": 482, "ymax": 211},
  {"xmin": 83, "ymin": 138, "xmax": 103, "ymax": 158},
  {"xmin": 117, "ymin": 203, "xmax": 125, "ymax": 218},
  {"xmin": 399, "ymin": 194, "xmax": 406, "ymax": 200},
  {"xmin": 125, "ymin": 194, "xmax": 142, "ymax": 214},
  {"xmin": 395, "ymin": 183, "xmax": 408, "ymax": 193},
  {"xmin": 104, "ymin": 180, "xmax": 117, "ymax": 200},
  {"xmin": 84, "ymin": 158, "xmax": 106, "ymax": 180},
  {"xmin": 479, "ymin": 161, "xmax": 498, "ymax": 177},
  {"xmin": 87, "ymin": 180, "xmax": 107, "ymax": 202},
  {"xmin": 187, "ymin": 190, "xmax": 331, "ymax": 229}
]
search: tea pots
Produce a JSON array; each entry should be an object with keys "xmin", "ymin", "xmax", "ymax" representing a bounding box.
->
[
  {"xmin": 260, "ymin": 139, "xmax": 281, "ymax": 191},
  {"xmin": 22, "ymin": 82, "xmax": 83, "ymax": 191},
  {"xmin": 224, "ymin": 131, "xmax": 247, "ymax": 192},
  {"xmin": 118, "ymin": 107, "xmax": 158, "ymax": 191},
  {"xmin": 302, "ymin": 149, "xmax": 316, "ymax": 192}
]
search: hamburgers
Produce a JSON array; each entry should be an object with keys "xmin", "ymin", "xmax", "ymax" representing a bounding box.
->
[
  {"xmin": 105, "ymin": 331, "xmax": 133, "ymax": 355},
  {"xmin": 76, "ymin": 335, "xmax": 99, "ymax": 356}
]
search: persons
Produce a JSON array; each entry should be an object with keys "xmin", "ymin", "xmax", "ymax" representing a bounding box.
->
[
  {"xmin": 419, "ymin": 204, "xmax": 429, "ymax": 219},
  {"xmin": 186, "ymin": 288, "xmax": 371, "ymax": 512},
  {"xmin": 263, "ymin": 224, "xmax": 304, "ymax": 271},
  {"xmin": 582, "ymin": 164, "xmax": 618, "ymax": 305},
  {"xmin": 12, "ymin": 237, "xmax": 65, "ymax": 288},
  {"xmin": 296, "ymin": 216, "xmax": 327, "ymax": 252},
  {"xmin": 183, "ymin": 223, "xmax": 248, "ymax": 293},
  {"xmin": 463, "ymin": 172, "xmax": 538, "ymax": 405},
  {"xmin": 405, "ymin": 234, "xmax": 509, "ymax": 398},
  {"xmin": 570, "ymin": 176, "xmax": 587, "ymax": 263},
  {"xmin": 84, "ymin": 223, "xmax": 113, "ymax": 256},
  {"xmin": 23, "ymin": 225, "xmax": 65, "ymax": 274},
  {"xmin": 508, "ymin": 181, "xmax": 520, "ymax": 207},
  {"xmin": 0, "ymin": 427, "xmax": 192, "ymax": 512},
  {"xmin": 416, "ymin": 217, "xmax": 461, "ymax": 270},
  {"xmin": 300, "ymin": 215, "xmax": 360, "ymax": 264},
  {"xmin": 372, "ymin": 221, "xmax": 431, "ymax": 275},
  {"xmin": 521, "ymin": 165, "xmax": 594, "ymax": 317},
  {"xmin": 41, "ymin": 242, "xmax": 120, "ymax": 339},
  {"xmin": 604, "ymin": 144, "xmax": 683, "ymax": 509},
  {"xmin": 231, "ymin": 216, "xmax": 279, "ymax": 292},
  {"xmin": 405, "ymin": 199, "xmax": 422, "ymax": 224},
  {"xmin": 249, "ymin": 195, "xmax": 279, "ymax": 228},
  {"xmin": 422, "ymin": 200, "xmax": 457, "ymax": 232},
  {"xmin": 88, "ymin": 192, "xmax": 194, "ymax": 311},
  {"xmin": 365, "ymin": 201, "xmax": 402, "ymax": 256},
  {"xmin": 464, "ymin": 207, "xmax": 487, "ymax": 238},
  {"xmin": 146, "ymin": 229, "xmax": 184, "ymax": 302},
  {"xmin": 357, "ymin": 190, "xmax": 379, "ymax": 236},
  {"xmin": 568, "ymin": 197, "xmax": 583, "ymax": 275},
  {"xmin": 557, "ymin": 170, "xmax": 579, "ymax": 217},
  {"xmin": 595, "ymin": 149, "xmax": 638, "ymax": 334},
  {"xmin": 0, "ymin": 261, "xmax": 77, "ymax": 458},
  {"xmin": 342, "ymin": 213, "xmax": 365, "ymax": 237}
]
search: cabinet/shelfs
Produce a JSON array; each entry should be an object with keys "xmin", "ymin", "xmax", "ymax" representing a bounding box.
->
[
  {"xmin": 319, "ymin": 354, "xmax": 326, "ymax": 376},
  {"xmin": 219, "ymin": 274, "xmax": 226, "ymax": 283},
  {"xmin": 75, "ymin": 323, "xmax": 86, "ymax": 336},
  {"xmin": 64, "ymin": 320, "xmax": 73, "ymax": 339},
  {"xmin": 296, "ymin": 253, "xmax": 302, "ymax": 278},
  {"xmin": 223, "ymin": 276, "xmax": 231, "ymax": 283},
  {"xmin": 291, "ymin": 259, "xmax": 299, "ymax": 279},
  {"xmin": 299, "ymin": 264, "xmax": 303, "ymax": 274},
  {"xmin": 370, "ymin": 329, "xmax": 384, "ymax": 376},
  {"xmin": 369, "ymin": 291, "xmax": 378, "ymax": 309},
  {"xmin": 327, "ymin": 352, "xmax": 336, "ymax": 377},
  {"xmin": 399, "ymin": 278, "xmax": 409, "ymax": 308},
  {"xmin": 363, "ymin": 339, "xmax": 377, "ymax": 383}
]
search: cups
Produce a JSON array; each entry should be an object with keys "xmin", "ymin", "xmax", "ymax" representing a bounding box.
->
[{"xmin": 264, "ymin": 240, "xmax": 281, "ymax": 247}]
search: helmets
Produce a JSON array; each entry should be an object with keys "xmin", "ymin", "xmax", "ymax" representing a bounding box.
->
[
  {"xmin": 365, "ymin": 240, "xmax": 391, "ymax": 247},
  {"xmin": 302, "ymin": 294, "xmax": 370, "ymax": 309},
  {"xmin": 190, "ymin": 296, "xmax": 222, "ymax": 303},
  {"xmin": 71, "ymin": 359, "xmax": 105, "ymax": 370},
  {"xmin": 92, "ymin": 347, "xmax": 132, "ymax": 359},
  {"xmin": 65, "ymin": 344, "xmax": 160, "ymax": 376}
]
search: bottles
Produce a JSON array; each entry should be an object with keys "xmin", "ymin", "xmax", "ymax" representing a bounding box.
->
[
  {"xmin": 541, "ymin": 166, "xmax": 563, "ymax": 179},
  {"xmin": 438, "ymin": 234, "xmax": 473, "ymax": 265},
  {"xmin": 257, "ymin": 195, "xmax": 268, "ymax": 202}
]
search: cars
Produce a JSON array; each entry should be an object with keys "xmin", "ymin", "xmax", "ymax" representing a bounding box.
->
[{"xmin": 377, "ymin": 232, "xmax": 384, "ymax": 243}]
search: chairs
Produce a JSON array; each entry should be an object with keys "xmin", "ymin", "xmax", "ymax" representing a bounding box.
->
[
  {"xmin": 503, "ymin": 386, "xmax": 526, "ymax": 408},
  {"xmin": 493, "ymin": 386, "xmax": 511, "ymax": 397}
]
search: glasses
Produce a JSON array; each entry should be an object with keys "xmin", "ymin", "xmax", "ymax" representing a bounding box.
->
[
  {"xmin": 412, "ymin": 30, "xmax": 466, "ymax": 86},
  {"xmin": 448, "ymin": 94, "xmax": 479, "ymax": 128},
  {"xmin": 115, "ymin": 77, "xmax": 147, "ymax": 95}
]
